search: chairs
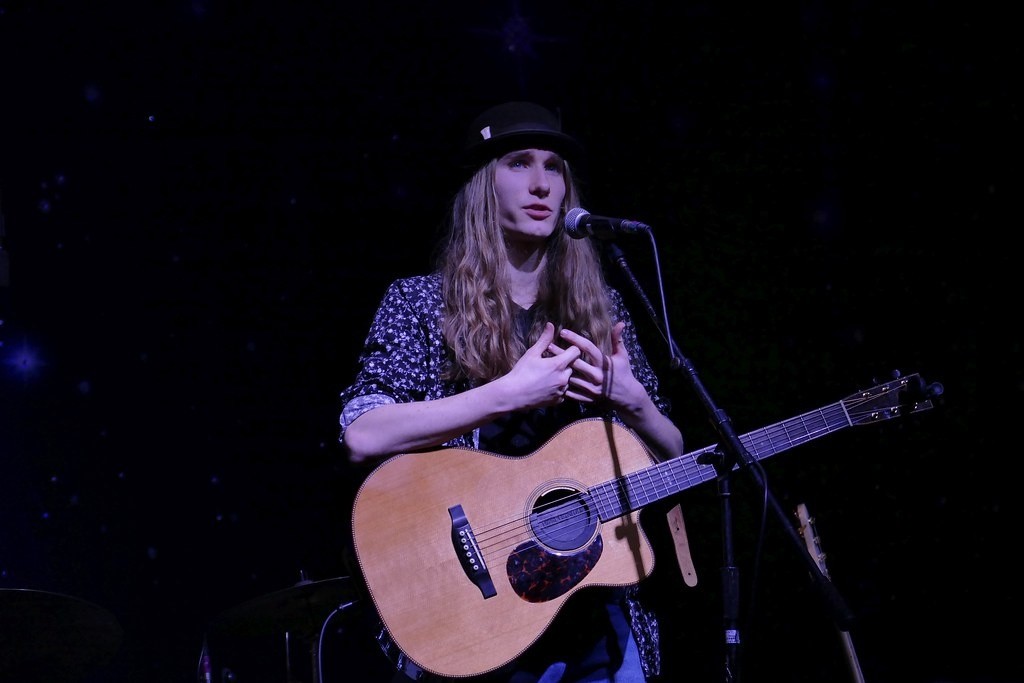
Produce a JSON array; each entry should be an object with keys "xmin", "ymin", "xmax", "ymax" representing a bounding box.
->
[
  {"xmin": 197, "ymin": 576, "xmax": 379, "ymax": 682},
  {"xmin": 0, "ymin": 588, "xmax": 125, "ymax": 683}
]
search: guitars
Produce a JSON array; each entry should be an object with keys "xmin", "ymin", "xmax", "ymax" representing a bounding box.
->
[
  {"xmin": 793, "ymin": 501, "xmax": 866, "ymax": 683},
  {"xmin": 349, "ymin": 369, "xmax": 946, "ymax": 679}
]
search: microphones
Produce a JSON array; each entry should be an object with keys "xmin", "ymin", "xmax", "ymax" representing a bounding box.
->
[
  {"xmin": 202, "ymin": 643, "xmax": 212, "ymax": 683},
  {"xmin": 564, "ymin": 208, "xmax": 651, "ymax": 239}
]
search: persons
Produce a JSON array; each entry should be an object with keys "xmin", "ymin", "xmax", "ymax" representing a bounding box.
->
[{"xmin": 339, "ymin": 98, "xmax": 683, "ymax": 683}]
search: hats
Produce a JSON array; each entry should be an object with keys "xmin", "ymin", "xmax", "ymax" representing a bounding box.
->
[{"xmin": 466, "ymin": 95, "xmax": 585, "ymax": 159}]
